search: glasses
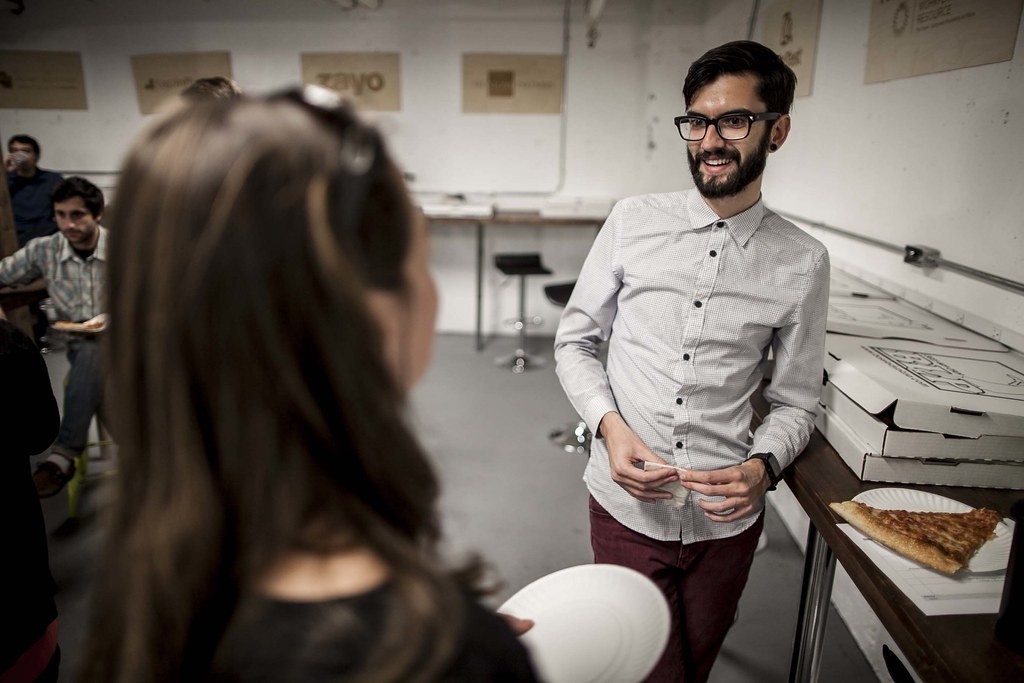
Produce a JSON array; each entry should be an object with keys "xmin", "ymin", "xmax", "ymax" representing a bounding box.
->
[
  {"xmin": 180, "ymin": 75, "xmax": 355, "ymax": 206},
  {"xmin": 674, "ymin": 113, "xmax": 783, "ymax": 140}
]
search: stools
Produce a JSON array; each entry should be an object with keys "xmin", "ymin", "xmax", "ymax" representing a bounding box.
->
[
  {"xmin": 490, "ymin": 251, "xmax": 553, "ymax": 368},
  {"xmin": 545, "ymin": 278, "xmax": 576, "ymax": 307},
  {"xmin": 62, "ymin": 367, "xmax": 109, "ymax": 531}
]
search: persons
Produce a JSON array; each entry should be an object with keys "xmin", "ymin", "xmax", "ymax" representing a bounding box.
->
[
  {"xmin": 36, "ymin": 83, "xmax": 543, "ymax": 683},
  {"xmin": 0, "ymin": 134, "xmax": 118, "ymax": 500},
  {"xmin": 0, "ymin": 319, "xmax": 62, "ymax": 683},
  {"xmin": 553, "ymin": 39, "xmax": 829, "ymax": 683},
  {"xmin": 180, "ymin": 77, "xmax": 239, "ymax": 106}
]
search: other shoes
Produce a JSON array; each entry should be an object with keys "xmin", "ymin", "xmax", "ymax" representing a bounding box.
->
[{"xmin": 33, "ymin": 458, "xmax": 75, "ymax": 497}]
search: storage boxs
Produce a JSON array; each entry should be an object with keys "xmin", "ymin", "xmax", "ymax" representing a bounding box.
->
[{"xmin": 764, "ymin": 268, "xmax": 1024, "ymax": 490}]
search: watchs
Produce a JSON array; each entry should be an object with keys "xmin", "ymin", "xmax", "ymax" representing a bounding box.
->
[{"xmin": 744, "ymin": 452, "xmax": 784, "ymax": 491}]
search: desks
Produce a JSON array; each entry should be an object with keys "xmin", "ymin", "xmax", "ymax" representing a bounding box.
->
[
  {"xmin": 423, "ymin": 214, "xmax": 605, "ymax": 349},
  {"xmin": 748, "ymin": 360, "xmax": 1023, "ymax": 682}
]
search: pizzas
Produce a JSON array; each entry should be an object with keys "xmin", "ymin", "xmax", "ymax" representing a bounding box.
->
[{"xmin": 827, "ymin": 500, "xmax": 1003, "ymax": 575}]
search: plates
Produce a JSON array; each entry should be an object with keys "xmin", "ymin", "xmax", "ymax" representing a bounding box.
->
[
  {"xmin": 848, "ymin": 487, "xmax": 1013, "ymax": 573},
  {"xmin": 49, "ymin": 323, "xmax": 101, "ymax": 332},
  {"xmin": 494, "ymin": 563, "xmax": 671, "ymax": 683}
]
